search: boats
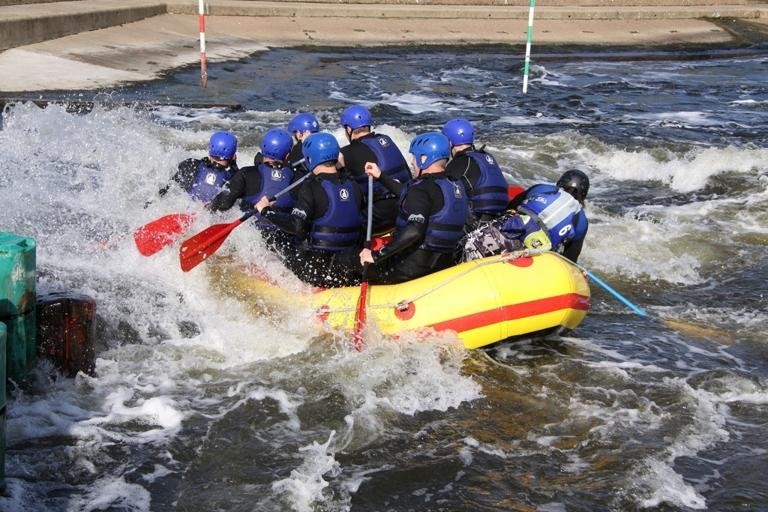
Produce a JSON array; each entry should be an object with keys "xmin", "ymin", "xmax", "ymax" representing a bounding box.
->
[{"xmin": 159, "ymin": 176, "xmax": 592, "ymax": 358}]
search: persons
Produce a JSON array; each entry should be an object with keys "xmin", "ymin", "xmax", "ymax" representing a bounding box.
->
[
  {"xmin": 253, "ymin": 130, "xmax": 363, "ymax": 287},
  {"xmin": 357, "ymin": 133, "xmax": 469, "ymax": 284},
  {"xmin": 285, "ymin": 112, "xmax": 319, "ymax": 170},
  {"xmin": 441, "ymin": 118, "xmax": 509, "ymax": 239},
  {"xmin": 453, "ymin": 169, "xmax": 589, "ymax": 265},
  {"xmin": 207, "ymin": 128, "xmax": 305, "ymax": 228},
  {"xmin": 338, "ymin": 105, "xmax": 413, "ymax": 235},
  {"xmin": 158, "ymin": 131, "xmax": 240, "ymax": 204}
]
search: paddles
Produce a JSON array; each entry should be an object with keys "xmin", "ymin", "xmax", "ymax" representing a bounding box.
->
[
  {"xmin": 574, "ymin": 264, "xmax": 730, "ymax": 345},
  {"xmin": 134, "ymin": 209, "xmax": 204, "ymax": 257},
  {"xmin": 179, "ymin": 173, "xmax": 311, "ymax": 273},
  {"xmin": 354, "ymin": 173, "xmax": 373, "ymax": 353}
]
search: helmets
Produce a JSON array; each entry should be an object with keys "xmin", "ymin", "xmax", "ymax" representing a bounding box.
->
[
  {"xmin": 209, "ymin": 133, "xmax": 237, "ymax": 160},
  {"xmin": 409, "ymin": 132, "xmax": 449, "ymax": 169},
  {"xmin": 302, "ymin": 133, "xmax": 339, "ymax": 170},
  {"xmin": 442, "ymin": 120, "xmax": 473, "ymax": 147},
  {"xmin": 288, "ymin": 114, "xmax": 318, "ymax": 133},
  {"xmin": 261, "ymin": 130, "xmax": 293, "ymax": 161},
  {"xmin": 341, "ymin": 105, "xmax": 372, "ymax": 129},
  {"xmin": 557, "ymin": 170, "xmax": 589, "ymax": 200}
]
